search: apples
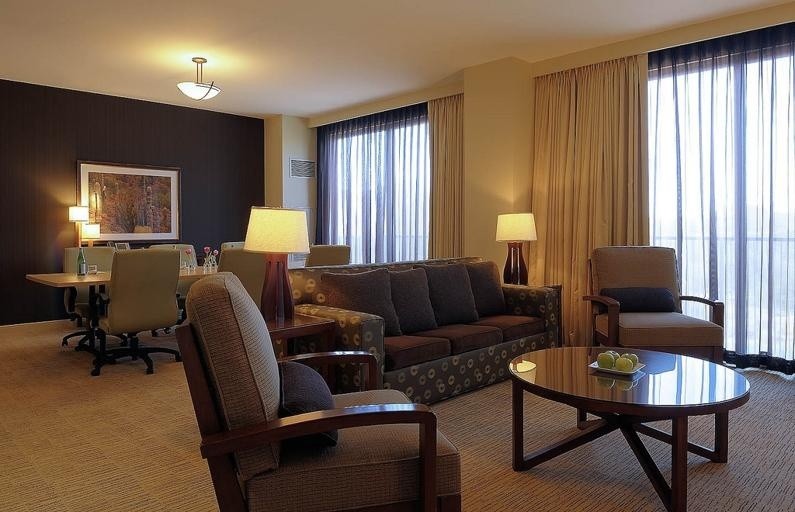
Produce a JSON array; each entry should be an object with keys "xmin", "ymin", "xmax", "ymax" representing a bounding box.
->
[{"xmin": 598, "ymin": 352, "xmax": 638, "ymax": 371}]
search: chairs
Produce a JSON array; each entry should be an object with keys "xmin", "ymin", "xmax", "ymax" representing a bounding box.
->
[
  {"xmin": 174, "ymin": 273, "xmax": 462, "ymax": 511},
  {"xmin": 62, "ymin": 241, "xmax": 351, "ymax": 376},
  {"xmin": 582, "ymin": 245, "xmax": 724, "ymax": 365}
]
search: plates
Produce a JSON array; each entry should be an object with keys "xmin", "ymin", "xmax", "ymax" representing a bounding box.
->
[
  {"xmin": 588, "ymin": 371, "xmax": 645, "ymax": 382},
  {"xmin": 588, "ymin": 361, "xmax": 646, "ymax": 375}
]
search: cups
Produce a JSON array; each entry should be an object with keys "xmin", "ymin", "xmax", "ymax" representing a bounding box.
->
[{"xmin": 88, "ymin": 264, "xmax": 98, "ymax": 274}]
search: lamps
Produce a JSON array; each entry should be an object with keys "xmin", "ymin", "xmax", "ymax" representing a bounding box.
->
[
  {"xmin": 245, "ymin": 206, "xmax": 309, "ymax": 321},
  {"xmin": 509, "ymin": 355, "xmax": 538, "ymax": 385},
  {"xmin": 177, "ymin": 57, "xmax": 220, "ymax": 102},
  {"xmin": 67, "ymin": 205, "xmax": 90, "ymax": 223},
  {"xmin": 82, "ymin": 223, "xmax": 100, "ymax": 240},
  {"xmin": 496, "ymin": 214, "xmax": 538, "ymax": 285}
]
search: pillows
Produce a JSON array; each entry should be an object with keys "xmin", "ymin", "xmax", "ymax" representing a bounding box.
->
[
  {"xmin": 424, "ymin": 264, "xmax": 479, "ymax": 326},
  {"xmin": 467, "ymin": 261, "xmax": 506, "ymax": 317},
  {"xmin": 321, "ymin": 268, "xmax": 403, "ymax": 336},
  {"xmin": 280, "ymin": 361, "xmax": 338, "ymax": 452},
  {"xmin": 600, "ymin": 287, "xmax": 675, "ymax": 313},
  {"xmin": 389, "ymin": 268, "xmax": 438, "ymax": 332}
]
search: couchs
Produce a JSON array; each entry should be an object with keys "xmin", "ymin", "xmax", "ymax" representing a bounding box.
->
[{"xmin": 287, "ymin": 256, "xmax": 559, "ymax": 406}]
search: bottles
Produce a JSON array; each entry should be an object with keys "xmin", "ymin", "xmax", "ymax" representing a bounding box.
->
[{"xmin": 77, "ymin": 247, "xmax": 86, "ymax": 277}]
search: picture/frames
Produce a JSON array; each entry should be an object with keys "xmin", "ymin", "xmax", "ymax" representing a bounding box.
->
[{"xmin": 76, "ymin": 160, "xmax": 182, "ymax": 244}]
